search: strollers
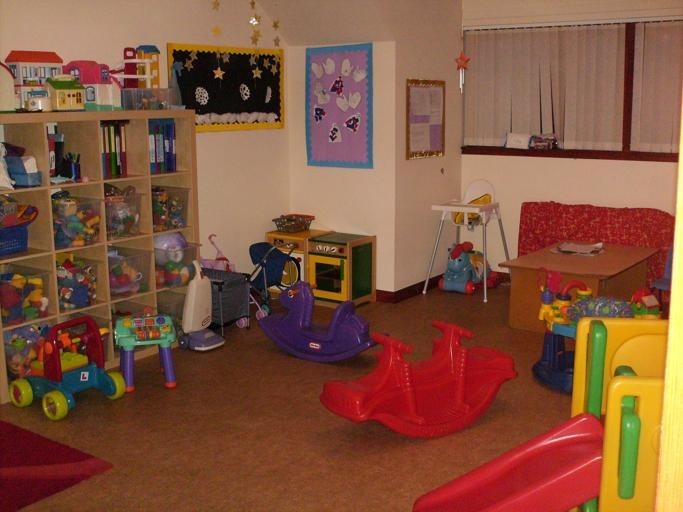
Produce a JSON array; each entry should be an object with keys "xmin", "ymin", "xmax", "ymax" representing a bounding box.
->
[
  {"xmin": 195, "ymin": 230, "xmax": 269, "ymax": 330},
  {"xmin": 246, "ymin": 238, "xmax": 303, "ymax": 312}
]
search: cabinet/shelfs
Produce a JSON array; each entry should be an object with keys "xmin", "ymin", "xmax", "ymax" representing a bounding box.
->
[{"xmin": 0, "ymin": 106, "xmax": 200, "ymax": 405}]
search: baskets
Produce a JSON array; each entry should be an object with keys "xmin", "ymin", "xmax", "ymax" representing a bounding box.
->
[
  {"xmin": 272, "ymin": 214, "xmax": 315, "ymax": 233},
  {"xmin": 0, "ymin": 226, "xmax": 28, "ymax": 256}
]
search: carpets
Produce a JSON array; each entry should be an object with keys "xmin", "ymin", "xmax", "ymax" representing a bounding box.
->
[{"xmin": 0, "ymin": 420, "xmax": 114, "ymax": 512}]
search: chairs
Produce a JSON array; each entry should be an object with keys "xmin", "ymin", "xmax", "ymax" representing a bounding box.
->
[
  {"xmin": 650, "ymin": 246, "xmax": 673, "ymax": 297},
  {"xmin": 423, "ymin": 179, "xmax": 512, "ymax": 303}
]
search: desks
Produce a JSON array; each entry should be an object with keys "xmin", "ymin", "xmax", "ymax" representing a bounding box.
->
[{"xmin": 498, "ymin": 240, "xmax": 661, "ymax": 334}]
